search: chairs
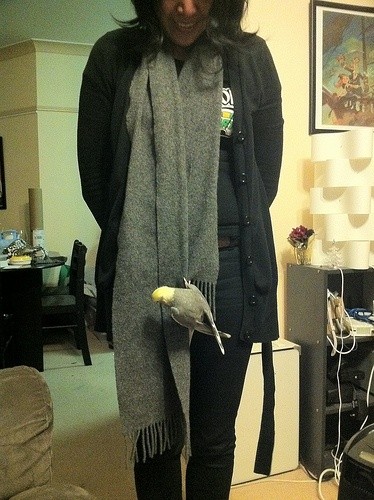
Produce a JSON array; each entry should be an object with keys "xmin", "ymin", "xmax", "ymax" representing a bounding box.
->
[
  {"xmin": 0, "ymin": 366, "xmax": 90, "ymax": 500},
  {"xmin": 43, "ymin": 240, "xmax": 79, "ymax": 296},
  {"xmin": 43, "ymin": 242, "xmax": 92, "ymax": 366}
]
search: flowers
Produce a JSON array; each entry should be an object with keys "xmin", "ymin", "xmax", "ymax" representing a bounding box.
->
[{"xmin": 288, "ymin": 225, "xmax": 312, "ymax": 245}]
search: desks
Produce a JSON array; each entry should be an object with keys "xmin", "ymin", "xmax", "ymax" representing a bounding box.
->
[
  {"xmin": 0, "ymin": 263, "xmax": 43, "ymax": 369},
  {"xmin": 230, "ymin": 337, "xmax": 301, "ymax": 485}
]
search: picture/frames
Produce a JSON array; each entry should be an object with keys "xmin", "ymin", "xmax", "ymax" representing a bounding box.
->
[
  {"xmin": 309, "ymin": 0, "xmax": 374, "ymax": 134},
  {"xmin": 0, "ymin": 136, "xmax": 6, "ymax": 210}
]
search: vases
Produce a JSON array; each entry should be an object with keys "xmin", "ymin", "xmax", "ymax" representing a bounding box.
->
[{"xmin": 294, "ymin": 245, "xmax": 313, "ymax": 267}]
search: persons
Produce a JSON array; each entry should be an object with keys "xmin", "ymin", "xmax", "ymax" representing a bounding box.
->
[{"xmin": 77, "ymin": 0, "xmax": 284, "ymax": 500}]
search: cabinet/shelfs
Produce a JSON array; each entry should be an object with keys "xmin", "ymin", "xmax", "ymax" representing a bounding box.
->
[{"xmin": 283, "ymin": 262, "xmax": 373, "ymax": 480}]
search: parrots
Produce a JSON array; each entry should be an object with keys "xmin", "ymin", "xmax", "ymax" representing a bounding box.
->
[{"xmin": 151, "ymin": 277, "xmax": 231, "ymax": 355}]
